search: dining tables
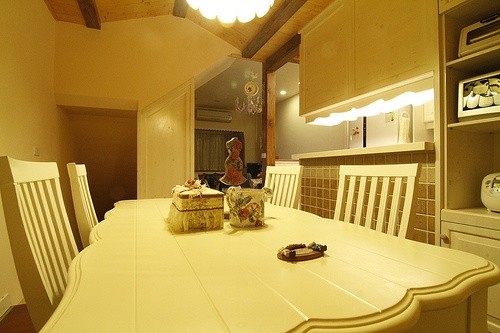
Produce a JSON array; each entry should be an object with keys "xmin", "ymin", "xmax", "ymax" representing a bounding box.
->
[{"xmin": 38, "ymin": 199, "xmax": 500, "ymax": 333}]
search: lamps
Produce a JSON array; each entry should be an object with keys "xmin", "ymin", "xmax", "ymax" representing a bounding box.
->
[{"xmin": 185, "ymin": 0, "xmax": 275, "ymax": 25}]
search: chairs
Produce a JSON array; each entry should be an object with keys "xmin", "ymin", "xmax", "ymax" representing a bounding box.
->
[
  {"xmin": 334, "ymin": 163, "xmax": 422, "ymax": 241},
  {"xmin": 66, "ymin": 162, "xmax": 99, "ymax": 249},
  {"xmin": 264, "ymin": 165, "xmax": 304, "ymax": 210},
  {"xmin": 0, "ymin": 155, "xmax": 80, "ymax": 333}
]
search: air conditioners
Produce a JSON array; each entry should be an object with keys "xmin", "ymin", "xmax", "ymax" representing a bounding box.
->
[{"xmin": 195, "ymin": 109, "xmax": 232, "ymax": 123}]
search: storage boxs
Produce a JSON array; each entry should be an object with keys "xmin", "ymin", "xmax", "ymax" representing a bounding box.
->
[{"xmin": 175, "ymin": 187, "xmax": 225, "ymax": 231}]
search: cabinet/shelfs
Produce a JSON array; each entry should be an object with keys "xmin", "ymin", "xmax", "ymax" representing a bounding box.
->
[
  {"xmin": 435, "ymin": 0, "xmax": 500, "ymax": 333},
  {"xmin": 299, "ymin": 0, "xmax": 436, "ymax": 125}
]
